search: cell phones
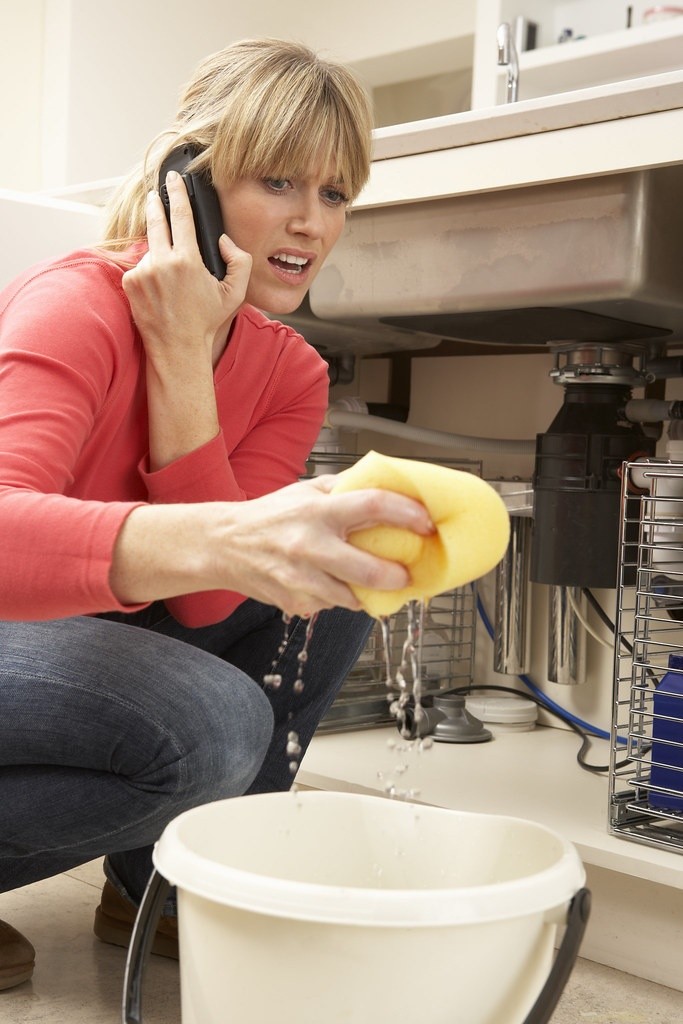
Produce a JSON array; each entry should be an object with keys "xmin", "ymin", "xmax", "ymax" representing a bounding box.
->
[{"xmin": 158, "ymin": 142, "xmax": 227, "ymax": 281}]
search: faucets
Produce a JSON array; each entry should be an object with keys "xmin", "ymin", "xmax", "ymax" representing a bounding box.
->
[{"xmin": 496, "ymin": 21, "xmax": 519, "ymax": 102}]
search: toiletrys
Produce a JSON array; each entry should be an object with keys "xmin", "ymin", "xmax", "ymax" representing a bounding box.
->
[{"xmin": 641, "ymin": 6, "xmax": 683, "ymax": 26}]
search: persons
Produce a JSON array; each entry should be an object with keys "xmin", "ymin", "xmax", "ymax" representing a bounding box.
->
[{"xmin": 0, "ymin": 37, "xmax": 436, "ymax": 1001}]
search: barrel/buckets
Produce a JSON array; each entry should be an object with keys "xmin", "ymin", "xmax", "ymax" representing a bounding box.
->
[{"xmin": 122, "ymin": 791, "xmax": 591, "ymax": 1024}]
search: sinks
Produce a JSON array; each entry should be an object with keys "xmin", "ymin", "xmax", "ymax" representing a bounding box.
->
[{"xmin": 309, "ymin": 69, "xmax": 683, "ymax": 347}]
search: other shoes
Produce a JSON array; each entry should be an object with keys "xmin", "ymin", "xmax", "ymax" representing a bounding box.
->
[
  {"xmin": 0, "ymin": 919, "xmax": 36, "ymax": 991},
  {"xmin": 91, "ymin": 879, "xmax": 179, "ymax": 962}
]
